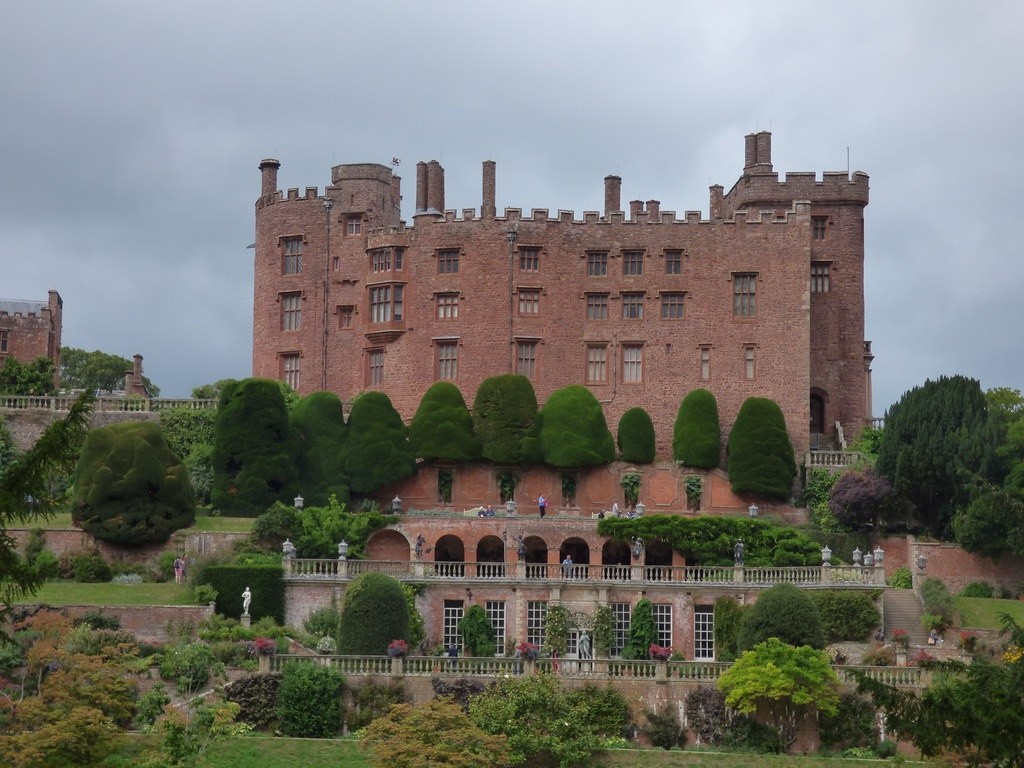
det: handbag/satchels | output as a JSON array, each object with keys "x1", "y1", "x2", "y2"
[{"x1": 544, "y1": 501, "x2": 550, "y2": 507}]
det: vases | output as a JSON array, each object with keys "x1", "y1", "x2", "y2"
[
  {"x1": 322, "y1": 651, "x2": 331, "y2": 655},
  {"x1": 965, "y1": 643, "x2": 975, "y2": 651},
  {"x1": 897, "y1": 639, "x2": 902, "y2": 644}
]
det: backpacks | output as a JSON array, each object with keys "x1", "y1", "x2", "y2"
[
  {"x1": 875, "y1": 633, "x2": 880, "y2": 640},
  {"x1": 175, "y1": 560, "x2": 180, "y2": 568}
]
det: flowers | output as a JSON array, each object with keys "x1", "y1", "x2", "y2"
[
  {"x1": 959, "y1": 631, "x2": 981, "y2": 648},
  {"x1": 317, "y1": 635, "x2": 336, "y2": 654},
  {"x1": 387, "y1": 639, "x2": 408, "y2": 656},
  {"x1": 255, "y1": 634, "x2": 275, "y2": 654},
  {"x1": 515, "y1": 640, "x2": 539, "y2": 659},
  {"x1": 891, "y1": 628, "x2": 911, "y2": 642},
  {"x1": 544, "y1": 604, "x2": 570, "y2": 656},
  {"x1": 591, "y1": 606, "x2": 616, "y2": 656},
  {"x1": 650, "y1": 643, "x2": 673, "y2": 661}
]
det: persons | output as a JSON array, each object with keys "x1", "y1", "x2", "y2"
[
  {"x1": 927, "y1": 629, "x2": 939, "y2": 645},
  {"x1": 598, "y1": 502, "x2": 636, "y2": 520},
  {"x1": 628, "y1": 535, "x2": 642, "y2": 560},
  {"x1": 577, "y1": 631, "x2": 592, "y2": 661},
  {"x1": 734, "y1": 538, "x2": 744, "y2": 566},
  {"x1": 478, "y1": 504, "x2": 487, "y2": 517},
  {"x1": 446, "y1": 643, "x2": 459, "y2": 671},
  {"x1": 874, "y1": 628, "x2": 885, "y2": 643},
  {"x1": 242, "y1": 586, "x2": 252, "y2": 614},
  {"x1": 173, "y1": 554, "x2": 190, "y2": 583},
  {"x1": 512, "y1": 534, "x2": 526, "y2": 560},
  {"x1": 560, "y1": 554, "x2": 574, "y2": 579},
  {"x1": 538, "y1": 492, "x2": 547, "y2": 519},
  {"x1": 487, "y1": 504, "x2": 496, "y2": 518},
  {"x1": 415, "y1": 533, "x2": 425, "y2": 557}
]
